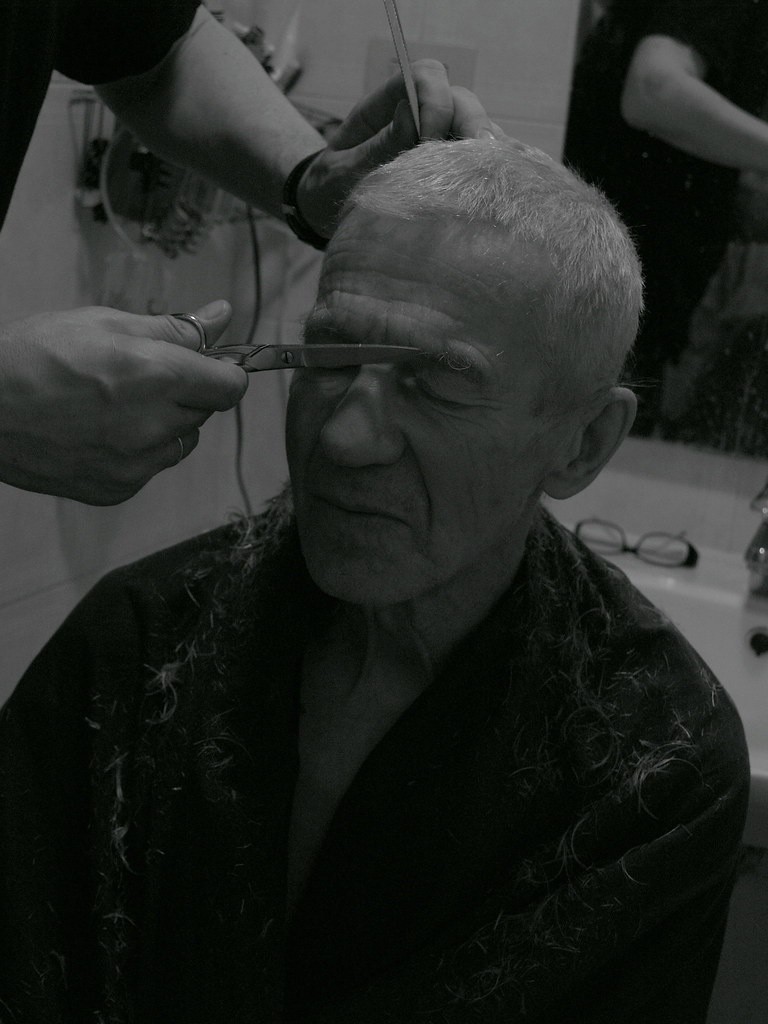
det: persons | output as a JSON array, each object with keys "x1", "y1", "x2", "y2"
[
  {"x1": 0, "y1": 0, "x2": 510, "y2": 507},
  {"x1": 564, "y1": 0, "x2": 768, "y2": 436},
  {"x1": 0, "y1": 137, "x2": 749, "y2": 1024}
]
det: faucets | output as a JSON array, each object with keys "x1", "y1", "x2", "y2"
[{"x1": 744, "y1": 480, "x2": 768, "y2": 606}]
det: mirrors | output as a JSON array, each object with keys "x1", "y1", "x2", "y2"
[{"x1": 562, "y1": 0, "x2": 767, "y2": 460}]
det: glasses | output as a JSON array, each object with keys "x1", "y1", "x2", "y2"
[{"x1": 574, "y1": 519, "x2": 698, "y2": 569}]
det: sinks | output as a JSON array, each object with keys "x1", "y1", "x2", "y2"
[{"x1": 550, "y1": 519, "x2": 768, "y2": 857}]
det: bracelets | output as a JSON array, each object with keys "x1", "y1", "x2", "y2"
[{"x1": 283, "y1": 149, "x2": 330, "y2": 251}]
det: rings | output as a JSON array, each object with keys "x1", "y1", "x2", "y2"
[{"x1": 171, "y1": 437, "x2": 183, "y2": 465}]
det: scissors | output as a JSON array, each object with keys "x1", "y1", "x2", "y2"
[{"x1": 166, "y1": 308, "x2": 433, "y2": 376}]
